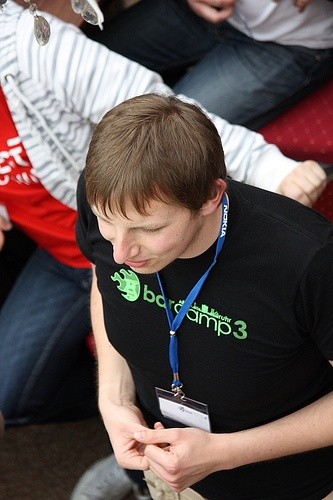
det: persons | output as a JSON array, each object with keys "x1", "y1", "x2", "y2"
[
  {"x1": 0, "y1": 0, "x2": 333, "y2": 424},
  {"x1": 70, "y1": 93, "x2": 333, "y2": 500}
]
